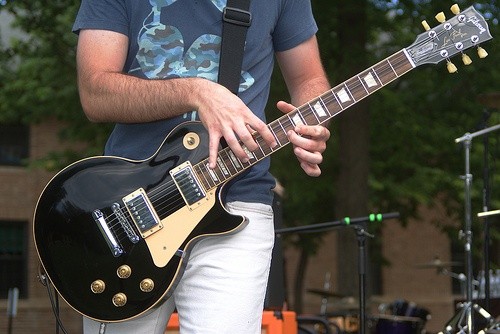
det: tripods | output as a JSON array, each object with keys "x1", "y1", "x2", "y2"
[{"x1": 437, "y1": 124, "x2": 500, "y2": 334}]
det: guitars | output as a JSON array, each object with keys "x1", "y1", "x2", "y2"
[{"x1": 32, "y1": 3, "x2": 493, "y2": 323}]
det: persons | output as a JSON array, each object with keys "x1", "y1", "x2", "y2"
[{"x1": 76, "y1": 0, "x2": 332, "y2": 334}]
